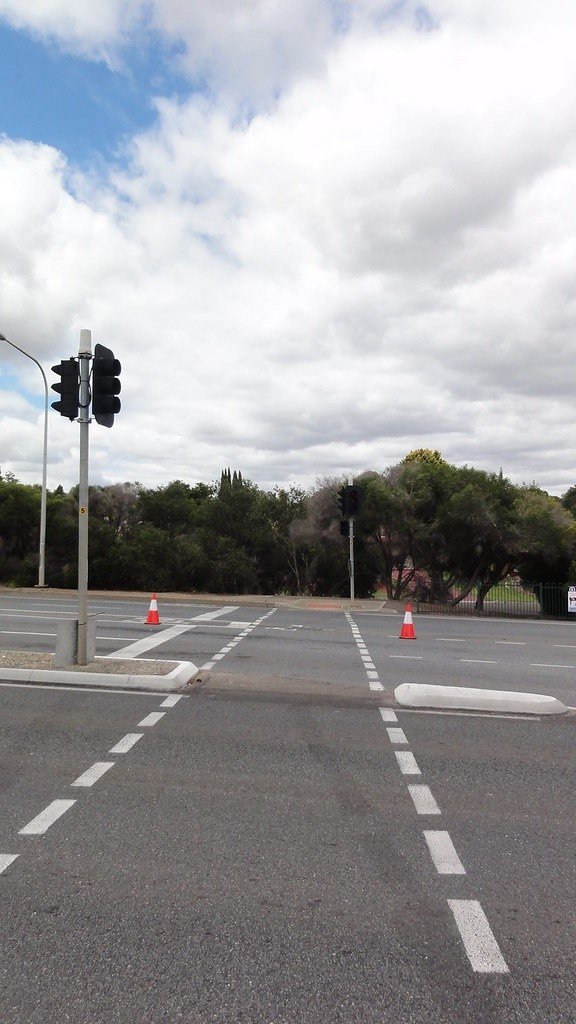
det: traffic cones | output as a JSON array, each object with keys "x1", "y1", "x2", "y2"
[
  {"x1": 399, "y1": 603, "x2": 416, "y2": 639},
  {"x1": 144, "y1": 593, "x2": 161, "y2": 624}
]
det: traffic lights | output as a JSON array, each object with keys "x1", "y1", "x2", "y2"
[
  {"x1": 51, "y1": 343, "x2": 122, "y2": 428},
  {"x1": 337, "y1": 484, "x2": 364, "y2": 517}
]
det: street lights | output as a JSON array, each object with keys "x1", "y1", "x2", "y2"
[{"x1": 0, "y1": 330, "x2": 49, "y2": 588}]
